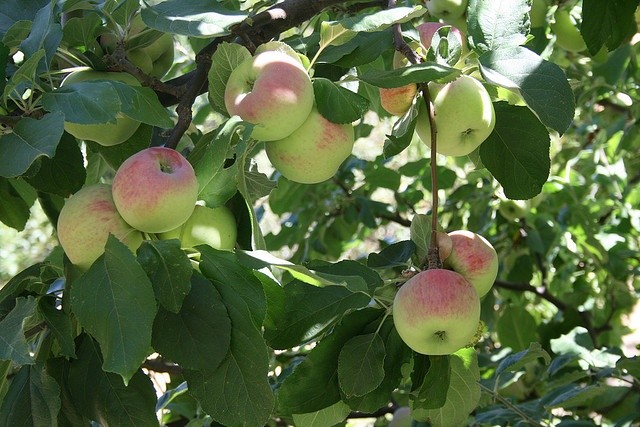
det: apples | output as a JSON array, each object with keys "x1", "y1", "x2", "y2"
[
  {"x1": 393, "y1": 21, "x2": 469, "y2": 70},
  {"x1": 414, "y1": 75, "x2": 496, "y2": 157},
  {"x1": 57, "y1": 68, "x2": 143, "y2": 146},
  {"x1": 57, "y1": 182, "x2": 135, "y2": 270},
  {"x1": 266, "y1": 107, "x2": 354, "y2": 184},
  {"x1": 99, "y1": 9, "x2": 175, "y2": 81},
  {"x1": 377, "y1": 86, "x2": 416, "y2": 115},
  {"x1": 392, "y1": 270, "x2": 481, "y2": 357},
  {"x1": 112, "y1": 147, "x2": 198, "y2": 235},
  {"x1": 226, "y1": 52, "x2": 316, "y2": 141},
  {"x1": 447, "y1": 230, "x2": 499, "y2": 299}
]
